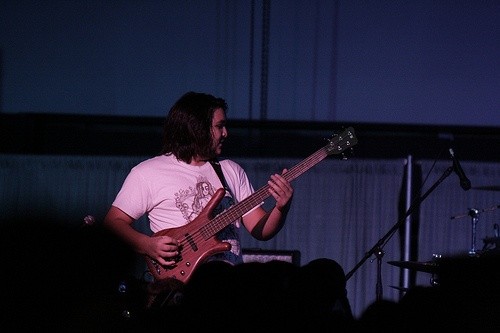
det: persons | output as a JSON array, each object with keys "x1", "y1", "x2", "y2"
[{"x1": 105, "y1": 92, "x2": 295, "y2": 271}]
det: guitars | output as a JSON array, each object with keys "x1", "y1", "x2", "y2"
[{"x1": 145, "y1": 124, "x2": 358, "y2": 283}]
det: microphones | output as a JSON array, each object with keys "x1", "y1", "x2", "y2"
[
  {"x1": 79, "y1": 215, "x2": 96, "y2": 227},
  {"x1": 449, "y1": 148, "x2": 471, "y2": 191}
]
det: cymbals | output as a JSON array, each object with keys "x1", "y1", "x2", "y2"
[{"x1": 387, "y1": 258, "x2": 440, "y2": 276}]
